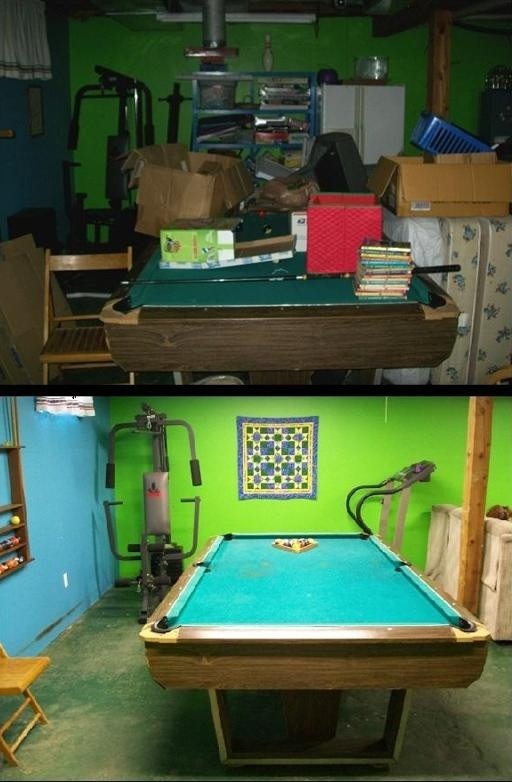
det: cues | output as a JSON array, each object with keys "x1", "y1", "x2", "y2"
[{"x1": 120, "y1": 263, "x2": 461, "y2": 286}]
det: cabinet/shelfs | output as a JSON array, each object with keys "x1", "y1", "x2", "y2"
[{"x1": 176, "y1": 74, "x2": 320, "y2": 175}]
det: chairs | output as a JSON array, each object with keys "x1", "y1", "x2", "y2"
[
  {"x1": 34, "y1": 245, "x2": 136, "y2": 386},
  {"x1": 0, "y1": 634, "x2": 52, "y2": 770}
]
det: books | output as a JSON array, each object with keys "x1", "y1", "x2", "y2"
[{"x1": 355, "y1": 240, "x2": 413, "y2": 302}]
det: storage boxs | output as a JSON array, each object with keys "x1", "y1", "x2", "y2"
[
  {"x1": 115, "y1": 145, "x2": 254, "y2": 237},
  {"x1": 366, "y1": 149, "x2": 512, "y2": 219}
]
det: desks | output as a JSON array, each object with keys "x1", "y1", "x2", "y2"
[
  {"x1": 102, "y1": 225, "x2": 466, "y2": 385},
  {"x1": 137, "y1": 531, "x2": 493, "y2": 772}
]
